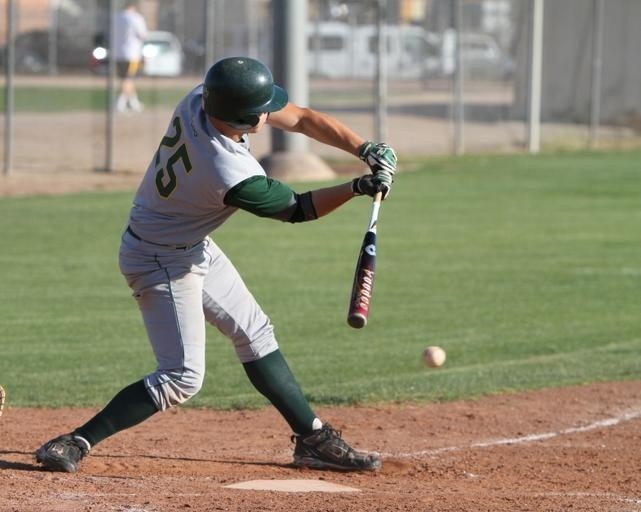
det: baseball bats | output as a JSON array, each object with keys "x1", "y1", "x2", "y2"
[{"x1": 347, "y1": 192, "x2": 382, "y2": 328}]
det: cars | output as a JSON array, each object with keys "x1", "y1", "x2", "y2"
[
  {"x1": 306, "y1": 19, "x2": 499, "y2": 78},
  {"x1": 0, "y1": 29, "x2": 102, "y2": 69},
  {"x1": 139, "y1": 32, "x2": 184, "y2": 78}
]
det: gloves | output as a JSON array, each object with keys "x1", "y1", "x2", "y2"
[
  {"x1": 349, "y1": 167, "x2": 392, "y2": 200},
  {"x1": 359, "y1": 139, "x2": 397, "y2": 174}
]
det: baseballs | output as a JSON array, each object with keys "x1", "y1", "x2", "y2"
[{"x1": 423, "y1": 346, "x2": 445, "y2": 367}]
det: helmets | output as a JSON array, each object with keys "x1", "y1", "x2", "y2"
[{"x1": 199, "y1": 54, "x2": 291, "y2": 131}]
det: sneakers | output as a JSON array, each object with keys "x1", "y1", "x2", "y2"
[
  {"x1": 289, "y1": 423, "x2": 381, "y2": 474},
  {"x1": 33, "y1": 429, "x2": 91, "y2": 474}
]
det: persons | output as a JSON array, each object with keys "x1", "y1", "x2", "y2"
[
  {"x1": 115, "y1": 1, "x2": 151, "y2": 117},
  {"x1": 34, "y1": 55, "x2": 397, "y2": 474}
]
